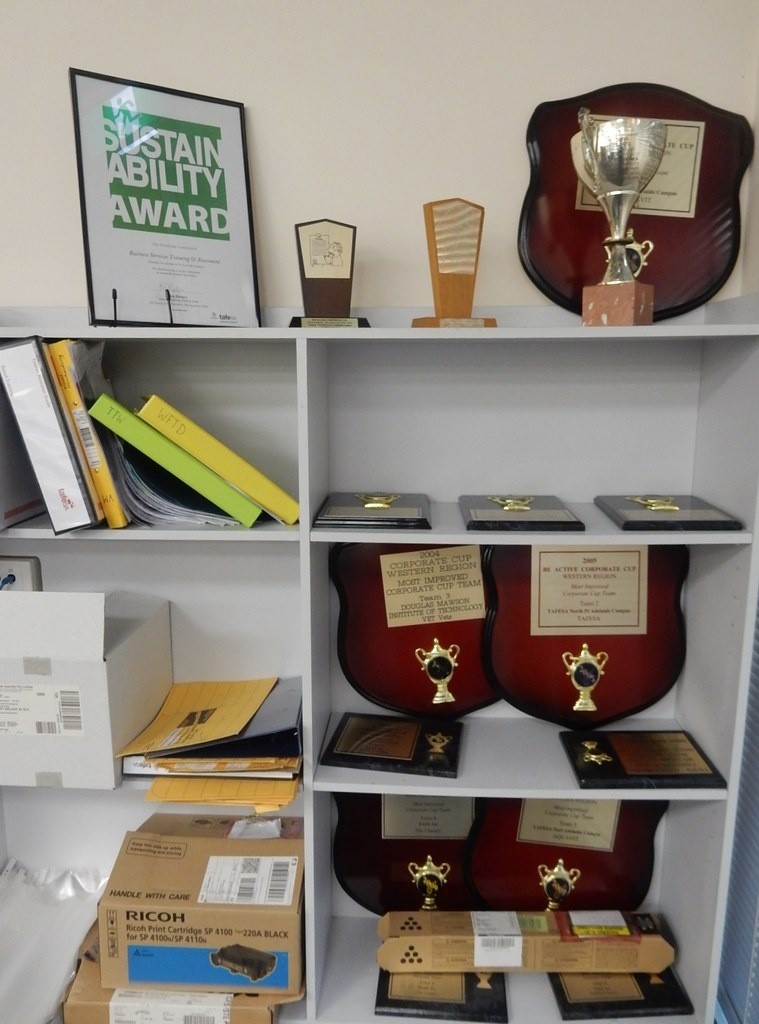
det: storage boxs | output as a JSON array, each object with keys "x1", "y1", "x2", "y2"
[
  {"x1": 98, "y1": 815, "x2": 305, "y2": 999},
  {"x1": 1, "y1": 590, "x2": 173, "y2": 790},
  {"x1": 1, "y1": 336, "x2": 105, "y2": 535},
  {"x1": 64, "y1": 922, "x2": 279, "y2": 1023},
  {"x1": 376, "y1": 910, "x2": 678, "y2": 972}
]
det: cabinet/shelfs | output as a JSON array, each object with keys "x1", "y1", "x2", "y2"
[{"x1": 0, "y1": 303, "x2": 758, "y2": 1024}]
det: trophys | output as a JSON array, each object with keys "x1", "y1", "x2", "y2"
[{"x1": 577, "y1": 107, "x2": 669, "y2": 328}]
